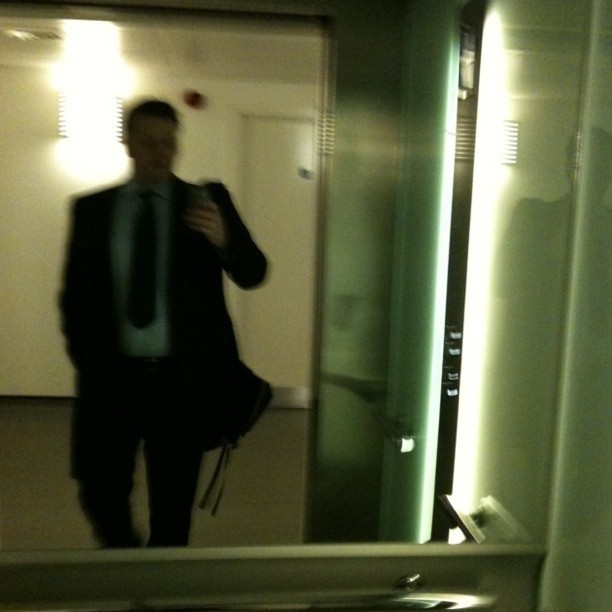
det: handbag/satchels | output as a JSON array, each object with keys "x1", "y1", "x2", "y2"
[{"x1": 168, "y1": 186, "x2": 272, "y2": 517}]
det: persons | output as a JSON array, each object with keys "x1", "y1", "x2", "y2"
[{"x1": 56, "y1": 99, "x2": 274, "y2": 545}]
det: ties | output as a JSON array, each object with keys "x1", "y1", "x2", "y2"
[{"x1": 124, "y1": 190, "x2": 156, "y2": 332}]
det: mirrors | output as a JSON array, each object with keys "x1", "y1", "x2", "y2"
[{"x1": 0, "y1": 0, "x2": 597, "y2": 551}]
